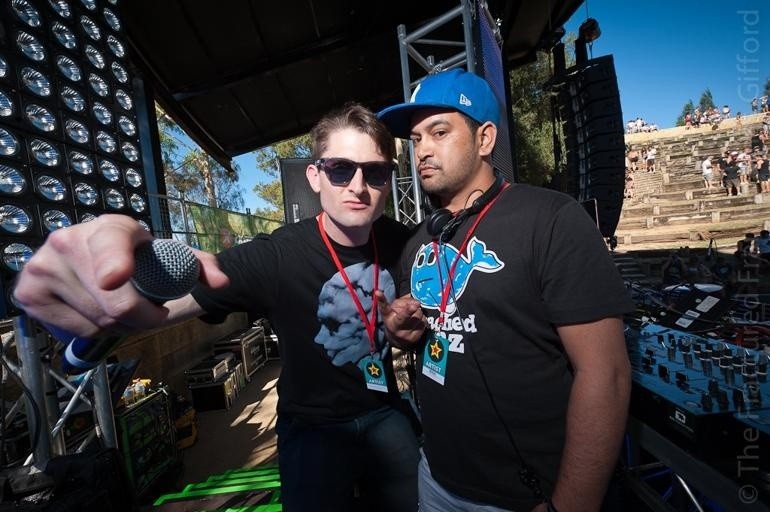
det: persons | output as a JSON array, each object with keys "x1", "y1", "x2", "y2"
[
  {"x1": 10, "y1": 99, "x2": 428, "y2": 512},
  {"x1": 618, "y1": 93, "x2": 770, "y2": 195},
  {"x1": 658, "y1": 230, "x2": 770, "y2": 313},
  {"x1": 374, "y1": 67, "x2": 645, "y2": 511}
]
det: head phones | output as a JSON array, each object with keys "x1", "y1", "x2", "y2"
[{"x1": 421, "y1": 166, "x2": 504, "y2": 239}]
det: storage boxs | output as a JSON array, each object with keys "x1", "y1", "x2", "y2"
[
  {"x1": 189, "y1": 361, "x2": 245, "y2": 413},
  {"x1": 184, "y1": 353, "x2": 237, "y2": 383},
  {"x1": 215, "y1": 327, "x2": 268, "y2": 382},
  {"x1": 263, "y1": 327, "x2": 281, "y2": 361}
]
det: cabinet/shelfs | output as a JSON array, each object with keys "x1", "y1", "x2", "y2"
[{"x1": 113, "y1": 384, "x2": 182, "y2": 506}]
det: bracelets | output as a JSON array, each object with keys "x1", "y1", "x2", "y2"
[{"x1": 546, "y1": 500, "x2": 560, "y2": 512}]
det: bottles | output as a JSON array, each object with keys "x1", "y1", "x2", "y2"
[
  {"x1": 124, "y1": 383, "x2": 137, "y2": 408},
  {"x1": 135, "y1": 378, "x2": 145, "y2": 401}
]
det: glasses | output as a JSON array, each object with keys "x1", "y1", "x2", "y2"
[{"x1": 311, "y1": 155, "x2": 403, "y2": 187}]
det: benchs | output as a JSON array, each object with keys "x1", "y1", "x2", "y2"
[{"x1": 622, "y1": 120, "x2": 769, "y2": 244}]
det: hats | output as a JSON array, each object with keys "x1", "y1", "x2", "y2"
[{"x1": 372, "y1": 66, "x2": 506, "y2": 134}]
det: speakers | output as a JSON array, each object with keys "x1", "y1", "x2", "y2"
[
  {"x1": 279, "y1": 158, "x2": 323, "y2": 224},
  {"x1": 553, "y1": 52, "x2": 627, "y2": 237}
]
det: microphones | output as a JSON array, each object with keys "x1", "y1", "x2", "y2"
[{"x1": 61, "y1": 240, "x2": 201, "y2": 376}]
